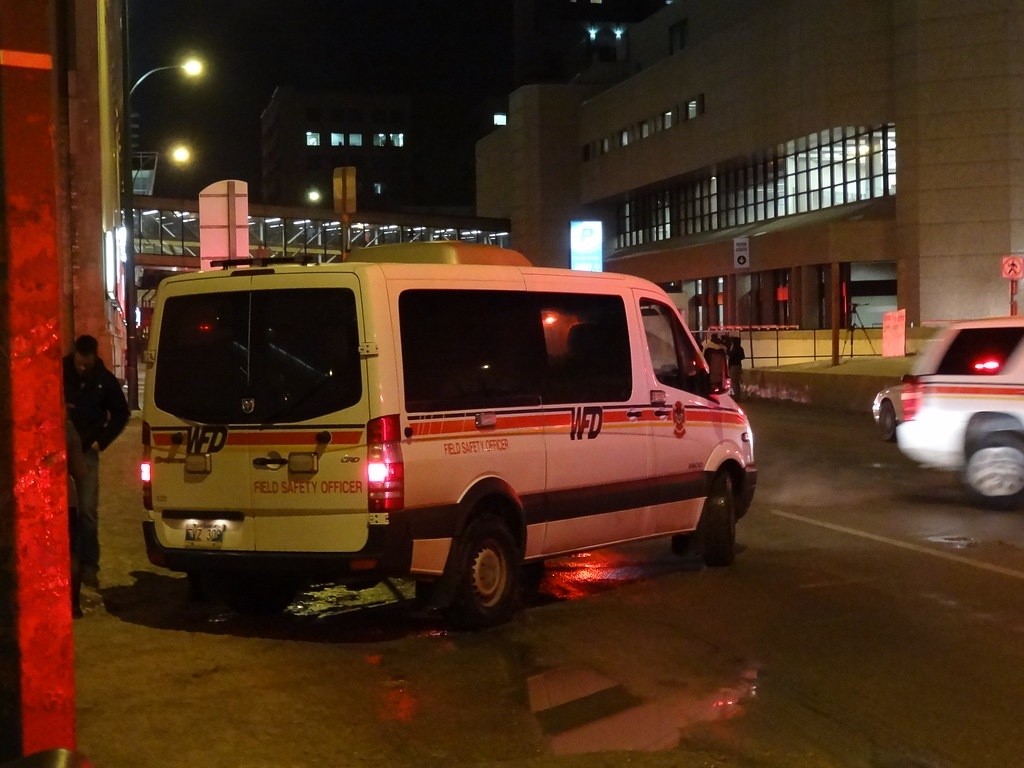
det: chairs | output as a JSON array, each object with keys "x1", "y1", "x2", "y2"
[{"x1": 549, "y1": 321, "x2": 631, "y2": 399}]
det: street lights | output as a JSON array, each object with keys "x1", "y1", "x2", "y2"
[{"x1": 124, "y1": 59, "x2": 200, "y2": 410}]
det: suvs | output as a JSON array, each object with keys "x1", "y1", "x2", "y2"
[{"x1": 874, "y1": 316, "x2": 1024, "y2": 443}]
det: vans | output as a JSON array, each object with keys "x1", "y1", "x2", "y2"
[{"x1": 142, "y1": 242, "x2": 757, "y2": 631}]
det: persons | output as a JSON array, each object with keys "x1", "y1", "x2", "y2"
[
  {"x1": 63, "y1": 334, "x2": 131, "y2": 616},
  {"x1": 702, "y1": 329, "x2": 745, "y2": 399}
]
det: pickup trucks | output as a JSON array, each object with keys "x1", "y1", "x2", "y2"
[{"x1": 897, "y1": 340, "x2": 1024, "y2": 510}]
